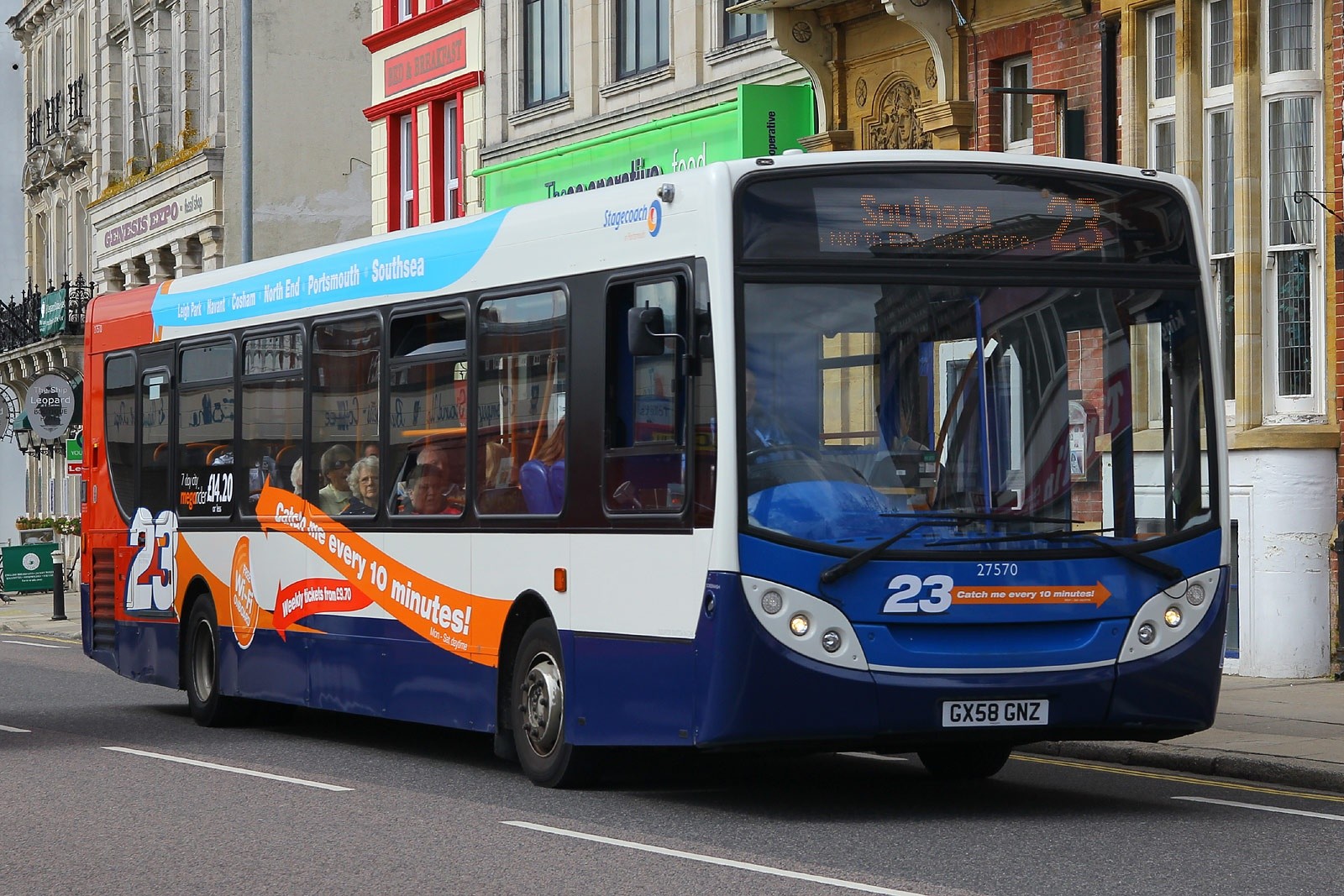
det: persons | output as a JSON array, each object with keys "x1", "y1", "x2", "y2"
[
  {"x1": 822, "y1": 399, "x2": 940, "y2": 511},
  {"x1": 694, "y1": 366, "x2": 815, "y2": 492},
  {"x1": 141, "y1": 415, "x2": 566, "y2": 515}
]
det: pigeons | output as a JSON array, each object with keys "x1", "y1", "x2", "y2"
[{"x1": 0, "y1": 593, "x2": 16, "y2": 605}]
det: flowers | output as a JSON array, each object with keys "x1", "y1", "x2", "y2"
[{"x1": 60, "y1": 520, "x2": 74, "y2": 534}]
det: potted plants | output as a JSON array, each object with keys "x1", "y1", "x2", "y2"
[{"x1": 15, "y1": 517, "x2": 81, "y2": 535}]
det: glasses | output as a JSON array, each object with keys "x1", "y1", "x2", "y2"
[
  {"x1": 358, "y1": 477, "x2": 379, "y2": 483},
  {"x1": 330, "y1": 460, "x2": 356, "y2": 470}
]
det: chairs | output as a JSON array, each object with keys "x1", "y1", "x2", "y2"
[{"x1": 151, "y1": 428, "x2": 525, "y2": 516}]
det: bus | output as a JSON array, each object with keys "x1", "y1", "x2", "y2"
[{"x1": 74, "y1": 145, "x2": 1232, "y2": 792}]
